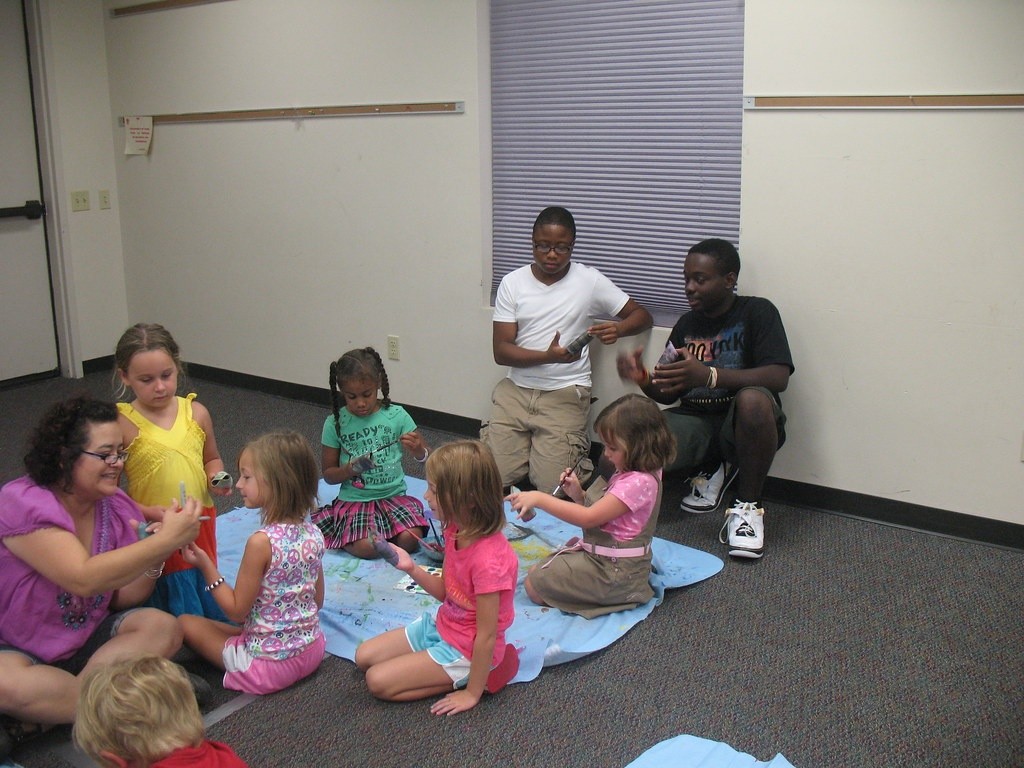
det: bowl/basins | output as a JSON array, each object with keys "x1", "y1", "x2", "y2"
[{"x1": 419, "y1": 536, "x2": 444, "y2": 563}]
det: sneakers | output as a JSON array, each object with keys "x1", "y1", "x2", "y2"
[
  {"x1": 679, "y1": 459, "x2": 740, "y2": 514},
  {"x1": 719, "y1": 497, "x2": 765, "y2": 558}
]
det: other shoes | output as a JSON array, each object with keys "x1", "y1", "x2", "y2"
[
  {"x1": 0, "y1": 714, "x2": 44, "y2": 762},
  {"x1": 482, "y1": 642, "x2": 519, "y2": 694},
  {"x1": 187, "y1": 673, "x2": 214, "y2": 706}
]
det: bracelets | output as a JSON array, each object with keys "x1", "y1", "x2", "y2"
[
  {"x1": 705, "y1": 366, "x2": 718, "y2": 389},
  {"x1": 204, "y1": 576, "x2": 225, "y2": 592},
  {"x1": 145, "y1": 562, "x2": 165, "y2": 578},
  {"x1": 414, "y1": 447, "x2": 429, "y2": 463}
]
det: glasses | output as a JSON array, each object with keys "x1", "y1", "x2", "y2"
[
  {"x1": 532, "y1": 238, "x2": 575, "y2": 254},
  {"x1": 80, "y1": 449, "x2": 130, "y2": 464}
]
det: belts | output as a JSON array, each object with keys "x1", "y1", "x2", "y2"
[{"x1": 541, "y1": 536, "x2": 655, "y2": 569}]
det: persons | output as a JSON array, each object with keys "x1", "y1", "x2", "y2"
[
  {"x1": 481, "y1": 205, "x2": 654, "y2": 499},
  {"x1": 177, "y1": 429, "x2": 326, "y2": 693},
  {"x1": 71, "y1": 650, "x2": 248, "y2": 768},
  {"x1": 0, "y1": 397, "x2": 202, "y2": 743},
  {"x1": 310, "y1": 347, "x2": 432, "y2": 560},
  {"x1": 616, "y1": 238, "x2": 796, "y2": 559},
  {"x1": 111, "y1": 322, "x2": 233, "y2": 623},
  {"x1": 355, "y1": 438, "x2": 518, "y2": 717},
  {"x1": 503, "y1": 393, "x2": 677, "y2": 619}
]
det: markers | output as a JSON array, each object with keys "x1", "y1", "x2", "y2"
[{"x1": 179, "y1": 480, "x2": 186, "y2": 508}]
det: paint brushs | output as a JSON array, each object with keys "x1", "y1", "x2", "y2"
[
  {"x1": 403, "y1": 526, "x2": 436, "y2": 550},
  {"x1": 427, "y1": 517, "x2": 441, "y2": 546},
  {"x1": 553, "y1": 449, "x2": 591, "y2": 497},
  {"x1": 374, "y1": 439, "x2": 400, "y2": 454}
]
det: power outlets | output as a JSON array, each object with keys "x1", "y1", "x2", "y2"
[
  {"x1": 387, "y1": 335, "x2": 400, "y2": 361},
  {"x1": 71, "y1": 190, "x2": 88, "y2": 212}
]
